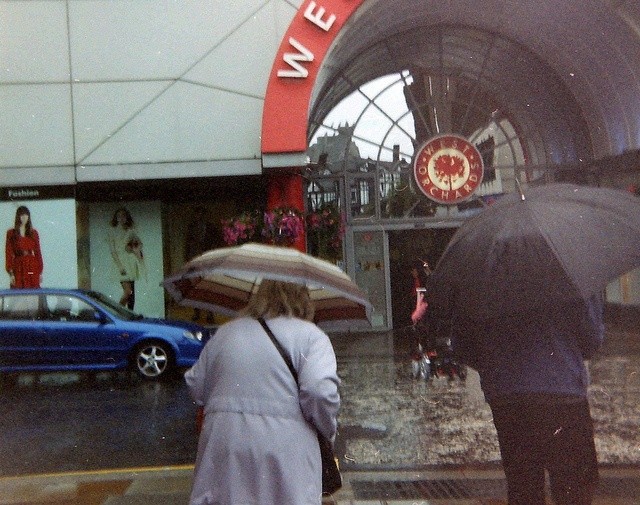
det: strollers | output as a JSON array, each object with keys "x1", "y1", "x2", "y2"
[{"x1": 412, "y1": 287, "x2": 467, "y2": 382}]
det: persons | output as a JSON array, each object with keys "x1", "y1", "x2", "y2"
[
  {"x1": 5, "y1": 204, "x2": 43, "y2": 289},
  {"x1": 451, "y1": 286, "x2": 607, "y2": 505},
  {"x1": 183, "y1": 277, "x2": 341, "y2": 505},
  {"x1": 106, "y1": 206, "x2": 145, "y2": 310}
]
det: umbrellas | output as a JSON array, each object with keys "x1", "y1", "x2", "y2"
[
  {"x1": 418, "y1": 176, "x2": 639, "y2": 324},
  {"x1": 159, "y1": 242, "x2": 376, "y2": 327}
]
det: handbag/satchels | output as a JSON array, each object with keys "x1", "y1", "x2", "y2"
[{"x1": 316, "y1": 431, "x2": 343, "y2": 498}]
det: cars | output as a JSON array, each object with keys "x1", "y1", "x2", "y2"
[{"x1": 0, "y1": 288, "x2": 210, "y2": 380}]
